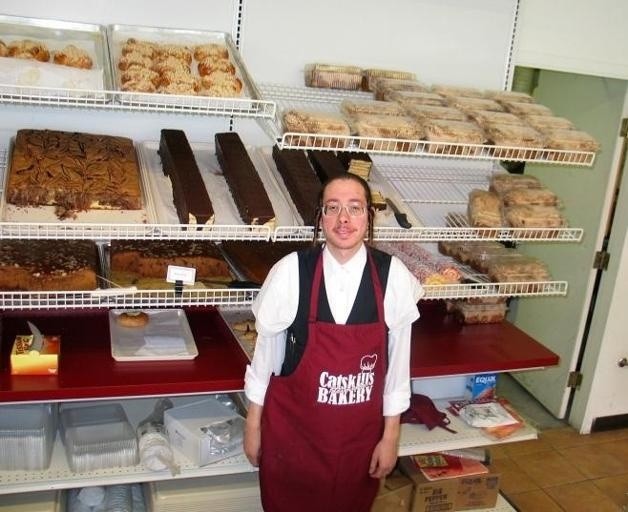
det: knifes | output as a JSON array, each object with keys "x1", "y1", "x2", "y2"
[{"x1": 386, "y1": 198, "x2": 411, "y2": 228}]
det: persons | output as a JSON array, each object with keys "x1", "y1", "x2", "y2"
[{"x1": 244, "y1": 172, "x2": 421, "y2": 512}]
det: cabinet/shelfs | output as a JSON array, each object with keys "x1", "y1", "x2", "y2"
[
  {"x1": 504, "y1": 66, "x2": 627, "y2": 435},
  {"x1": 1, "y1": 82, "x2": 596, "y2": 494}
]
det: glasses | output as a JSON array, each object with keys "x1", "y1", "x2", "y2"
[{"x1": 320, "y1": 202, "x2": 369, "y2": 217}]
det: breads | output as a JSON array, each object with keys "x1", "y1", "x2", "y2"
[{"x1": 0, "y1": 37, "x2": 601, "y2": 357}]
